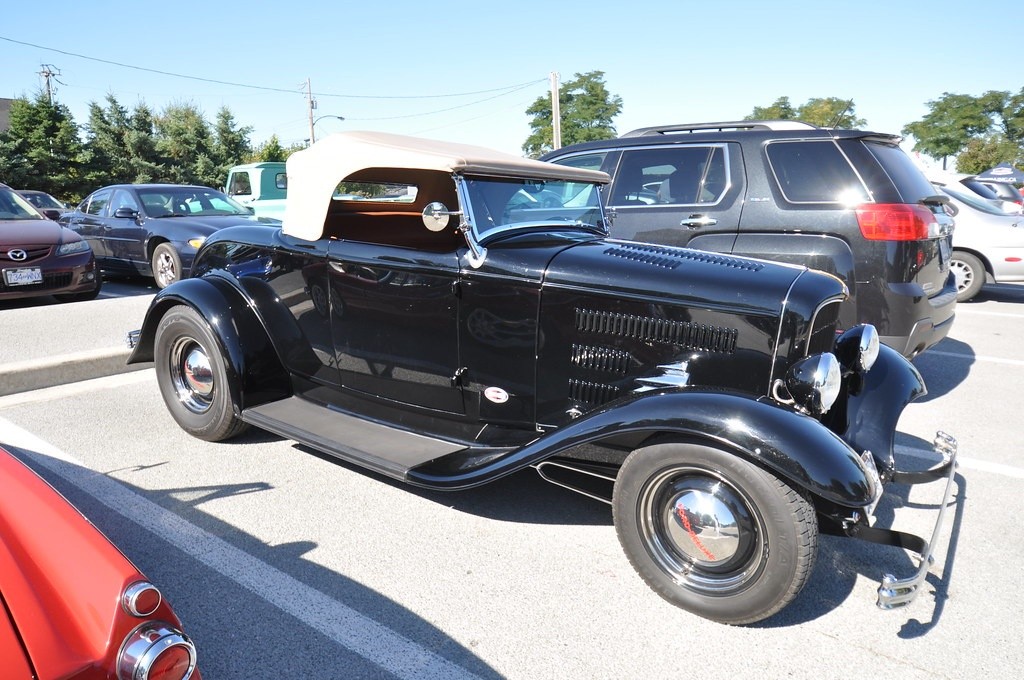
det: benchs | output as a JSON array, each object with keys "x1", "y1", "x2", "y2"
[{"x1": 326, "y1": 210, "x2": 465, "y2": 253}]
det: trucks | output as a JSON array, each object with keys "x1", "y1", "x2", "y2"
[{"x1": 225, "y1": 162, "x2": 416, "y2": 227}]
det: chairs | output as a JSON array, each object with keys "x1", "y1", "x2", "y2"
[
  {"x1": 670, "y1": 169, "x2": 696, "y2": 203},
  {"x1": 145, "y1": 204, "x2": 168, "y2": 217},
  {"x1": 621, "y1": 168, "x2": 648, "y2": 205}
]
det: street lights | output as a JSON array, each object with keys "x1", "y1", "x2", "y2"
[{"x1": 309, "y1": 115, "x2": 345, "y2": 144}]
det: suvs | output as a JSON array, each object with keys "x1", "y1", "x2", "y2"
[{"x1": 501, "y1": 120, "x2": 959, "y2": 360}]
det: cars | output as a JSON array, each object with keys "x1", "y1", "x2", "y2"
[
  {"x1": 0, "y1": 446, "x2": 202, "y2": 680},
  {"x1": 14, "y1": 190, "x2": 71, "y2": 217},
  {"x1": 55, "y1": 184, "x2": 271, "y2": 289},
  {"x1": 929, "y1": 174, "x2": 1024, "y2": 303},
  {"x1": 0, "y1": 183, "x2": 102, "y2": 301},
  {"x1": 126, "y1": 133, "x2": 960, "y2": 626}
]
